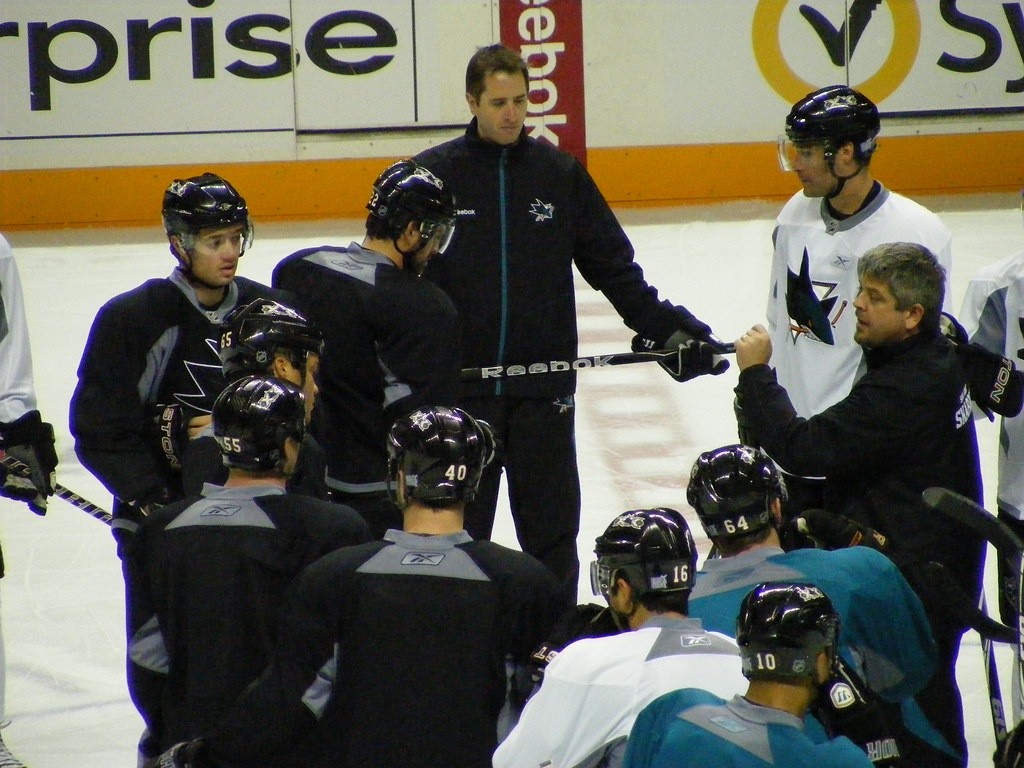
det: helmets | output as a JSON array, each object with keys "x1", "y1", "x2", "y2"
[
  {"x1": 160, "y1": 172, "x2": 252, "y2": 233},
  {"x1": 594, "y1": 507, "x2": 698, "y2": 598},
  {"x1": 367, "y1": 157, "x2": 457, "y2": 228},
  {"x1": 784, "y1": 84, "x2": 880, "y2": 164},
  {"x1": 220, "y1": 298, "x2": 332, "y2": 385},
  {"x1": 686, "y1": 444, "x2": 789, "y2": 546},
  {"x1": 211, "y1": 374, "x2": 309, "y2": 470},
  {"x1": 735, "y1": 579, "x2": 840, "y2": 688},
  {"x1": 385, "y1": 405, "x2": 499, "y2": 501}
]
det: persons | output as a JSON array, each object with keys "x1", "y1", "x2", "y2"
[
  {"x1": 764, "y1": 84, "x2": 957, "y2": 551},
  {"x1": 0, "y1": 232, "x2": 60, "y2": 768},
  {"x1": 409, "y1": 44, "x2": 728, "y2": 606},
  {"x1": 69, "y1": 156, "x2": 1024, "y2": 767}
]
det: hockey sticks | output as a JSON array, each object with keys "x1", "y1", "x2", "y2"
[
  {"x1": 0, "y1": 448, "x2": 116, "y2": 524},
  {"x1": 919, "y1": 485, "x2": 1024, "y2": 767},
  {"x1": 456, "y1": 340, "x2": 741, "y2": 380},
  {"x1": 974, "y1": 583, "x2": 1006, "y2": 755}
]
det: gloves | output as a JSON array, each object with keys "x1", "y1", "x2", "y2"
[
  {"x1": 126, "y1": 484, "x2": 191, "y2": 518},
  {"x1": 631, "y1": 303, "x2": 730, "y2": 382},
  {"x1": 529, "y1": 604, "x2": 630, "y2": 687},
  {"x1": 809, "y1": 647, "x2": 901, "y2": 767},
  {"x1": 791, "y1": 509, "x2": 890, "y2": 558},
  {"x1": 0, "y1": 409, "x2": 60, "y2": 517},
  {"x1": 950, "y1": 333, "x2": 1024, "y2": 418}
]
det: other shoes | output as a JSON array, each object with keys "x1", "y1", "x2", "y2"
[{"x1": 0, "y1": 722, "x2": 26, "y2": 768}]
